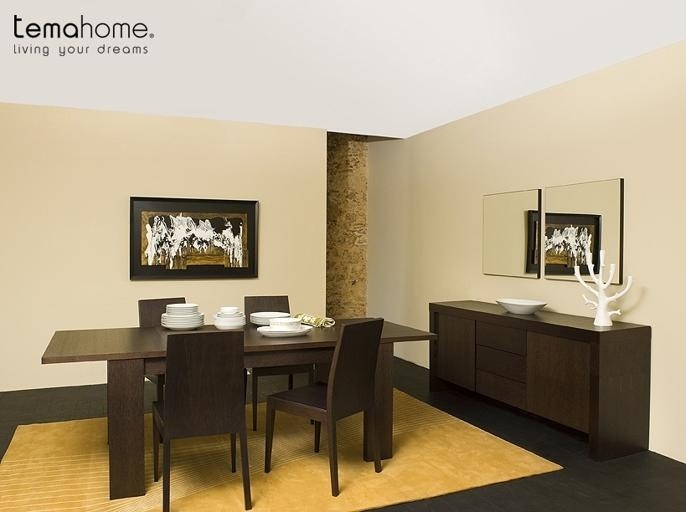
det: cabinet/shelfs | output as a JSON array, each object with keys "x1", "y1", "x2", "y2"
[{"x1": 429, "y1": 301, "x2": 651, "y2": 459}]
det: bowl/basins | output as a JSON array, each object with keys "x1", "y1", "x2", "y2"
[
  {"x1": 220, "y1": 306, "x2": 239, "y2": 314},
  {"x1": 496, "y1": 298, "x2": 548, "y2": 315}
]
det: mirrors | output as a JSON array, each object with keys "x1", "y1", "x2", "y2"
[
  {"x1": 545, "y1": 177, "x2": 622, "y2": 286},
  {"x1": 482, "y1": 190, "x2": 541, "y2": 279}
]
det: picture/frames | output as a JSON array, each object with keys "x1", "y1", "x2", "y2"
[
  {"x1": 129, "y1": 197, "x2": 259, "y2": 281},
  {"x1": 527, "y1": 210, "x2": 601, "y2": 275}
]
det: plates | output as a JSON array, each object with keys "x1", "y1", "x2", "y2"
[
  {"x1": 250, "y1": 311, "x2": 291, "y2": 326},
  {"x1": 213, "y1": 312, "x2": 247, "y2": 331},
  {"x1": 257, "y1": 325, "x2": 313, "y2": 337},
  {"x1": 161, "y1": 303, "x2": 204, "y2": 331},
  {"x1": 270, "y1": 317, "x2": 302, "y2": 326}
]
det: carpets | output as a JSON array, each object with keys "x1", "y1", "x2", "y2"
[{"x1": 0, "y1": 387, "x2": 564, "y2": 512}]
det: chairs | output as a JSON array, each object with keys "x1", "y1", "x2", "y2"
[
  {"x1": 245, "y1": 295, "x2": 314, "y2": 431},
  {"x1": 138, "y1": 297, "x2": 186, "y2": 397},
  {"x1": 152, "y1": 330, "x2": 252, "y2": 512},
  {"x1": 264, "y1": 316, "x2": 385, "y2": 497}
]
